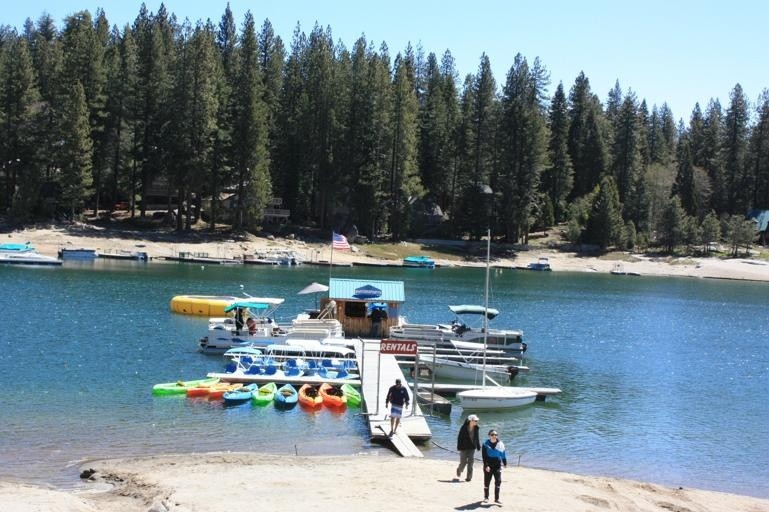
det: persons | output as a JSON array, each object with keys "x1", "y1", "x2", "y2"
[
  {"x1": 386, "y1": 379, "x2": 410, "y2": 434},
  {"x1": 457, "y1": 414, "x2": 480, "y2": 481},
  {"x1": 379, "y1": 307, "x2": 388, "y2": 339},
  {"x1": 367, "y1": 309, "x2": 380, "y2": 338},
  {"x1": 246, "y1": 318, "x2": 256, "y2": 336},
  {"x1": 235, "y1": 308, "x2": 244, "y2": 336},
  {"x1": 482, "y1": 430, "x2": 507, "y2": 507}
]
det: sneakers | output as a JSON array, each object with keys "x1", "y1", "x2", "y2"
[{"x1": 457, "y1": 469, "x2": 461, "y2": 477}]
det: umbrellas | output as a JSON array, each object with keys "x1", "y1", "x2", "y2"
[{"x1": 297, "y1": 282, "x2": 329, "y2": 312}]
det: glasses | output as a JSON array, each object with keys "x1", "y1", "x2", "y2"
[{"x1": 490, "y1": 433, "x2": 497, "y2": 436}]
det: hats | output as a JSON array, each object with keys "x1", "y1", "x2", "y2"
[{"x1": 468, "y1": 415, "x2": 479, "y2": 421}]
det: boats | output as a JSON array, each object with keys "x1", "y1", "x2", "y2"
[
  {"x1": 0, "y1": 242, "x2": 62, "y2": 265},
  {"x1": 389, "y1": 305, "x2": 526, "y2": 356},
  {"x1": 413, "y1": 385, "x2": 452, "y2": 417},
  {"x1": 195, "y1": 297, "x2": 343, "y2": 353},
  {"x1": 611, "y1": 269, "x2": 640, "y2": 277},
  {"x1": 527, "y1": 257, "x2": 550, "y2": 272},
  {"x1": 242, "y1": 251, "x2": 300, "y2": 265},
  {"x1": 170, "y1": 294, "x2": 247, "y2": 314},
  {"x1": 454, "y1": 388, "x2": 537, "y2": 410},
  {"x1": 402, "y1": 256, "x2": 435, "y2": 269},
  {"x1": 151, "y1": 344, "x2": 365, "y2": 410},
  {"x1": 408, "y1": 338, "x2": 530, "y2": 384}
]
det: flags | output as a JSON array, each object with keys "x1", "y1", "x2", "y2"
[{"x1": 332, "y1": 231, "x2": 351, "y2": 250}]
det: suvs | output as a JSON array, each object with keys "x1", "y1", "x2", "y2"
[{"x1": 114, "y1": 202, "x2": 128, "y2": 210}]
